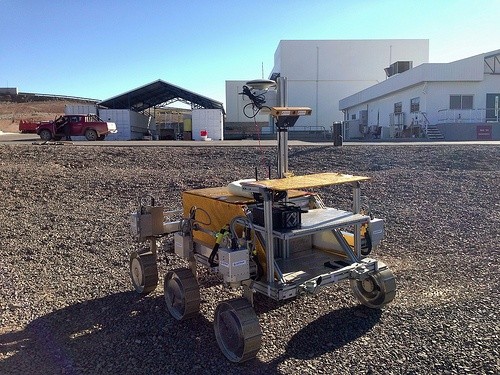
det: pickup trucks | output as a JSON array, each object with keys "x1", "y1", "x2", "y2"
[{"x1": 36, "y1": 113, "x2": 117, "y2": 141}]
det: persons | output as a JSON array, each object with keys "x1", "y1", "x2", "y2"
[{"x1": 61, "y1": 117, "x2": 72, "y2": 141}]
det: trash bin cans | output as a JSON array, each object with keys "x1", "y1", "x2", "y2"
[{"x1": 332, "y1": 120, "x2": 343, "y2": 146}]
